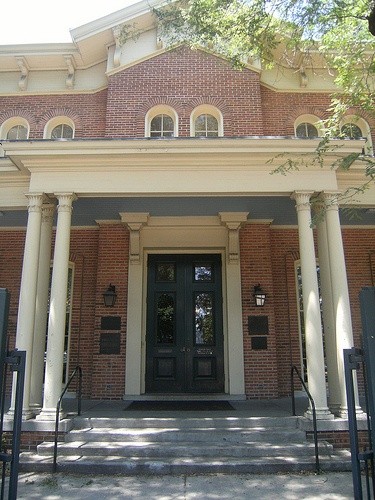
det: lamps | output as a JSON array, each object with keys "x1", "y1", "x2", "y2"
[
  {"x1": 253, "y1": 283, "x2": 266, "y2": 307},
  {"x1": 103, "y1": 284, "x2": 116, "y2": 307}
]
